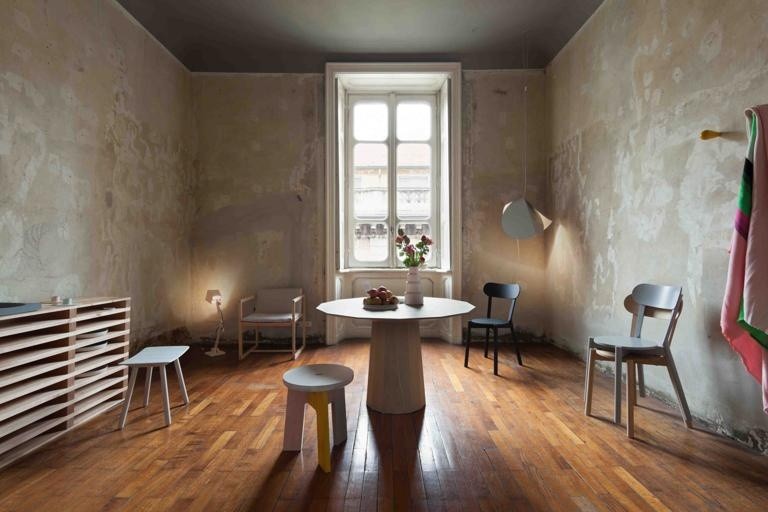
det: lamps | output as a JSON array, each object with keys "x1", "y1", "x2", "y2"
[
  {"x1": 205, "y1": 289, "x2": 227, "y2": 358},
  {"x1": 501, "y1": 84, "x2": 553, "y2": 240}
]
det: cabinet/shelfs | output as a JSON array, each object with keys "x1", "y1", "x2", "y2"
[{"x1": 0, "y1": 295, "x2": 133, "y2": 468}]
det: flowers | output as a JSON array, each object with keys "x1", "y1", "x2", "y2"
[{"x1": 396, "y1": 228, "x2": 434, "y2": 267}]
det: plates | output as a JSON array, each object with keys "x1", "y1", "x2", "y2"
[{"x1": 364, "y1": 305, "x2": 397, "y2": 310}]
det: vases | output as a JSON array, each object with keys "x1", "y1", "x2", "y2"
[{"x1": 404, "y1": 268, "x2": 423, "y2": 306}]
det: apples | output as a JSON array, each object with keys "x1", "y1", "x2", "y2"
[{"x1": 363, "y1": 286, "x2": 398, "y2": 305}]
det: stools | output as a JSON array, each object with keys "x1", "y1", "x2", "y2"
[
  {"x1": 118, "y1": 345, "x2": 190, "y2": 430},
  {"x1": 282, "y1": 363, "x2": 354, "y2": 474}
]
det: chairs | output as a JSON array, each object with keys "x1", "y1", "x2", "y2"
[
  {"x1": 464, "y1": 282, "x2": 522, "y2": 375},
  {"x1": 238, "y1": 289, "x2": 307, "y2": 361},
  {"x1": 584, "y1": 283, "x2": 693, "y2": 439}
]
